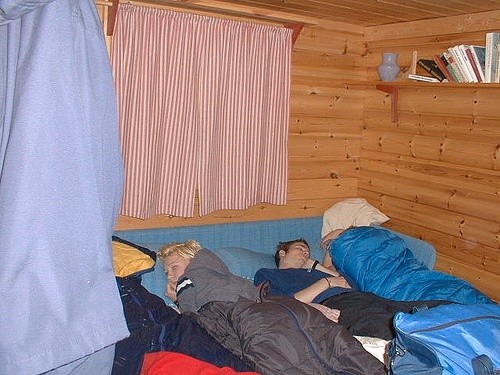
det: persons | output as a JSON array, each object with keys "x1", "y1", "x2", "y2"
[
  {"x1": 0, "y1": 1, "x2": 131, "y2": 375},
  {"x1": 156, "y1": 241, "x2": 261, "y2": 314},
  {"x1": 253, "y1": 226, "x2": 355, "y2": 304}
]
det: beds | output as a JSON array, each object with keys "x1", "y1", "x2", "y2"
[{"x1": 113, "y1": 214, "x2": 437, "y2": 312}]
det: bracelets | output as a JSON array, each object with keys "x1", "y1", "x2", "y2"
[
  {"x1": 325, "y1": 277, "x2": 331, "y2": 287},
  {"x1": 173, "y1": 300, "x2": 178, "y2": 304},
  {"x1": 312, "y1": 261, "x2": 319, "y2": 271}
]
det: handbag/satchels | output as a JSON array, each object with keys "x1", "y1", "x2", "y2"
[{"x1": 387, "y1": 303, "x2": 500, "y2": 375}]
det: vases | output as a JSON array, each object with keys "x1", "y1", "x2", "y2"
[{"x1": 376, "y1": 52, "x2": 401, "y2": 83}]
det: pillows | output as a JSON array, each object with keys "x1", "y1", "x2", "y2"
[{"x1": 211, "y1": 246, "x2": 278, "y2": 281}]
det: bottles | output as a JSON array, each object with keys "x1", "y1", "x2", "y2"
[{"x1": 377, "y1": 52, "x2": 401, "y2": 82}]
